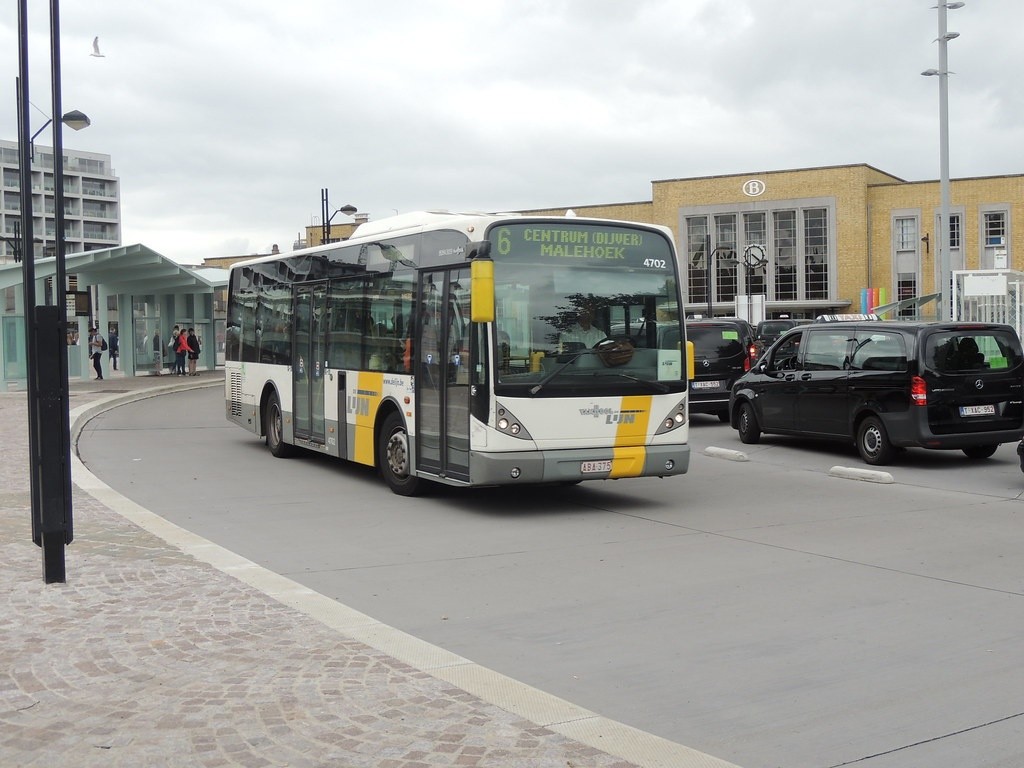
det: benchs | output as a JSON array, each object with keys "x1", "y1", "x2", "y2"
[{"x1": 138, "y1": 361, "x2": 178, "y2": 375}]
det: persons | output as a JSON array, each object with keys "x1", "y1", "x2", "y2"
[
  {"x1": 234, "y1": 304, "x2": 471, "y2": 383},
  {"x1": 65, "y1": 326, "x2": 204, "y2": 377},
  {"x1": 556, "y1": 298, "x2": 608, "y2": 354}
]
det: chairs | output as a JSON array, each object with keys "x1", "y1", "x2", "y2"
[
  {"x1": 232, "y1": 297, "x2": 379, "y2": 330},
  {"x1": 958, "y1": 337, "x2": 985, "y2": 367}
]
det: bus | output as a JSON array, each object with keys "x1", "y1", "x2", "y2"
[{"x1": 226, "y1": 212, "x2": 694, "y2": 497}]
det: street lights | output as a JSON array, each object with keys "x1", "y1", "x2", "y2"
[
  {"x1": 921, "y1": 0, "x2": 965, "y2": 323},
  {"x1": 321, "y1": 188, "x2": 357, "y2": 246},
  {"x1": 15, "y1": 0, "x2": 91, "y2": 583},
  {"x1": 706, "y1": 234, "x2": 730, "y2": 318}
]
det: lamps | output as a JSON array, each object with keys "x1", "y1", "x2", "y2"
[{"x1": 921, "y1": 233, "x2": 930, "y2": 253}]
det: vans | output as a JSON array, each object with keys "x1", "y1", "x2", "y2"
[{"x1": 730, "y1": 324, "x2": 1024, "y2": 465}]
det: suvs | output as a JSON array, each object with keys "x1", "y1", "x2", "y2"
[{"x1": 611, "y1": 314, "x2": 883, "y2": 423}]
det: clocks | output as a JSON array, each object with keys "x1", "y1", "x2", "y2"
[{"x1": 741, "y1": 243, "x2": 766, "y2": 270}]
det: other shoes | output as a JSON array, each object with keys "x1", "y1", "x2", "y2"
[
  {"x1": 156, "y1": 371, "x2": 200, "y2": 376},
  {"x1": 95, "y1": 377, "x2": 103, "y2": 380}
]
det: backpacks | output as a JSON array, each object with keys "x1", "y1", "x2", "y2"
[
  {"x1": 173, "y1": 336, "x2": 182, "y2": 350},
  {"x1": 95, "y1": 333, "x2": 108, "y2": 350}
]
